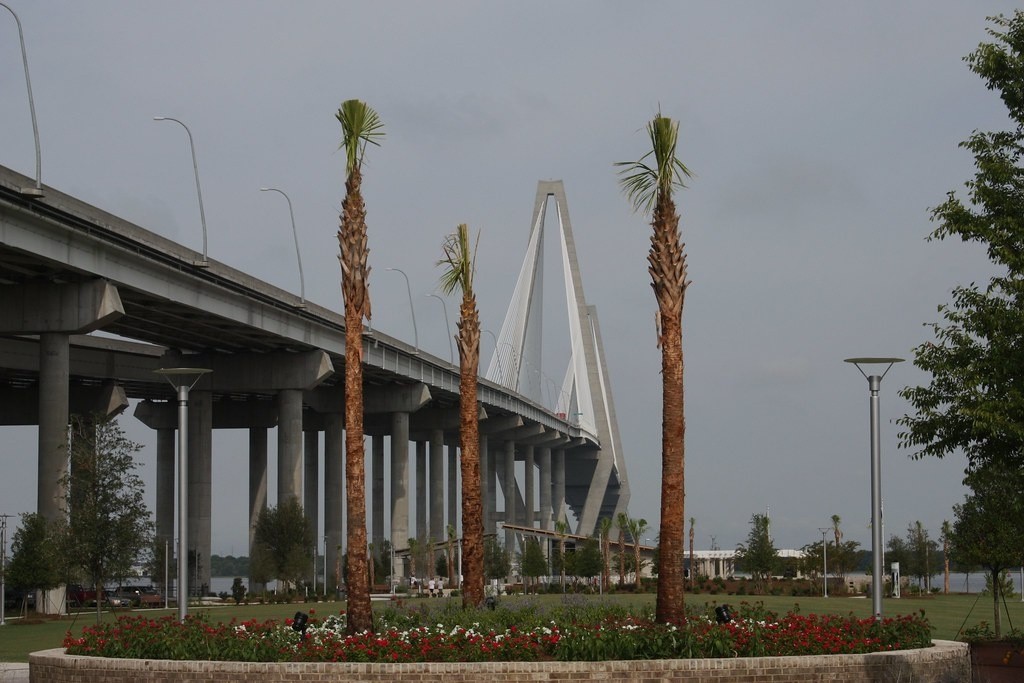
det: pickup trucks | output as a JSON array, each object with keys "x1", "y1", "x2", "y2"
[{"x1": 66, "y1": 584, "x2": 97, "y2": 606}]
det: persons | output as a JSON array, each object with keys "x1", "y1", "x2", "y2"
[
  {"x1": 428, "y1": 577, "x2": 436, "y2": 596},
  {"x1": 436, "y1": 577, "x2": 444, "y2": 593}
]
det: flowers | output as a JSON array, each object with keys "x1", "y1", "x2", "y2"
[{"x1": 960, "y1": 620, "x2": 1024, "y2": 664}]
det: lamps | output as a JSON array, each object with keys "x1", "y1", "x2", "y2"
[
  {"x1": 715, "y1": 607, "x2": 731, "y2": 623},
  {"x1": 293, "y1": 612, "x2": 308, "y2": 631},
  {"x1": 487, "y1": 597, "x2": 495, "y2": 610}
]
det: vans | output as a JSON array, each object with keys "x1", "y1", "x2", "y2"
[{"x1": 115, "y1": 586, "x2": 163, "y2": 607}]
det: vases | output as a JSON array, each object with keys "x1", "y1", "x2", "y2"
[{"x1": 970, "y1": 641, "x2": 1024, "y2": 683}]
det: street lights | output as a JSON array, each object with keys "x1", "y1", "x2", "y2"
[
  {"x1": 320, "y1": 535, "x2": 329, "y2": 595},
  {"x1": 482, "y1": 329, "x2": 504, "y2": 386},
  {"x1": 152, "y1": 368, "x2": 213, "y2": 622},
  {"x1": 386, "y1": 268, "x2": 419, "y2": 351},
  {"x1": 505, "y1": 342, "x2": 572, "y2": 420},
  {"x1": 425, "y1": 294, "x2": 453, "y2": 362},
  {"x1": 845, "y1": 357, "x2": 906, "y2": 620},
  {"x1": 819, "y1": 528, "x2": 831, "y2": 597},
  {"x1": 261, "y1": 187, "x2": 305, "y2": 303},
  {"x1": 0, "y1": 514, "x2": 15, "y2": 624},
  {"x1": 154, "y1": 116, "x2": 207, "y2": 262}
]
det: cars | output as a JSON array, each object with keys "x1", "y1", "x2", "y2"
[{"x1": 97, "y1": 590, "x2": 139, "y2": 608}]
